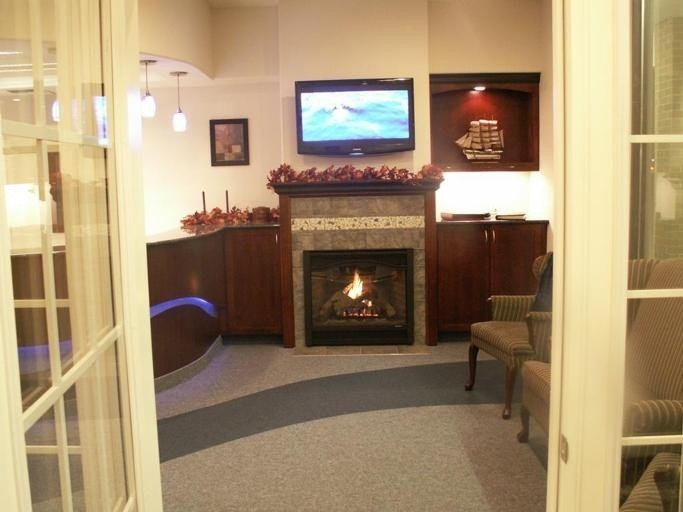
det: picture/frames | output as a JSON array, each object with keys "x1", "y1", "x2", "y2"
[
  {"x1": 431, "y1": 72, "x2": 541, "y2": 171},
  {"x1": 210, "y1": 119, "x2": 249, "y2": 165}
]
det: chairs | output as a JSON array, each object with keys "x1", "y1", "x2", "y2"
[
  {"x1": 465, "y1": 253, "x2": 551, "y2": 418},
  {"x1": 518, "y1": 258, "x2": 683, "y2": 485}
]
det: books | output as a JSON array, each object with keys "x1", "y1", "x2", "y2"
[{"x1": 440, "y1": 213, "x2": 528, "y2": 221}]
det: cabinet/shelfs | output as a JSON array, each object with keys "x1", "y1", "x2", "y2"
[
  {"x1": 438, "y1": 219, "x2": 549, "y2": 332},
  {"x1": 148, "y1": 215, "x2": 281, "y2": 387}
]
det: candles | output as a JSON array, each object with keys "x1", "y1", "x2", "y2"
[
  {"x1": 202, "y1": 190, "x2": 206, "y2": 212},
  {"x1": 226, "y1": 189, "x2": 230, "y2": 212}
]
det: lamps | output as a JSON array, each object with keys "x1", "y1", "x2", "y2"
[
  {"x1": 141, "y1": 59, "x2": 158, "y2": 117},
  {"x1": 170, "y1": 71, "x2": 188, "y2": 133}
]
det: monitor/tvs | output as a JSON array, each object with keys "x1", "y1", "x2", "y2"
[{"x1": 295, "y1": 78, "x2": 415, "y2": 156}]
